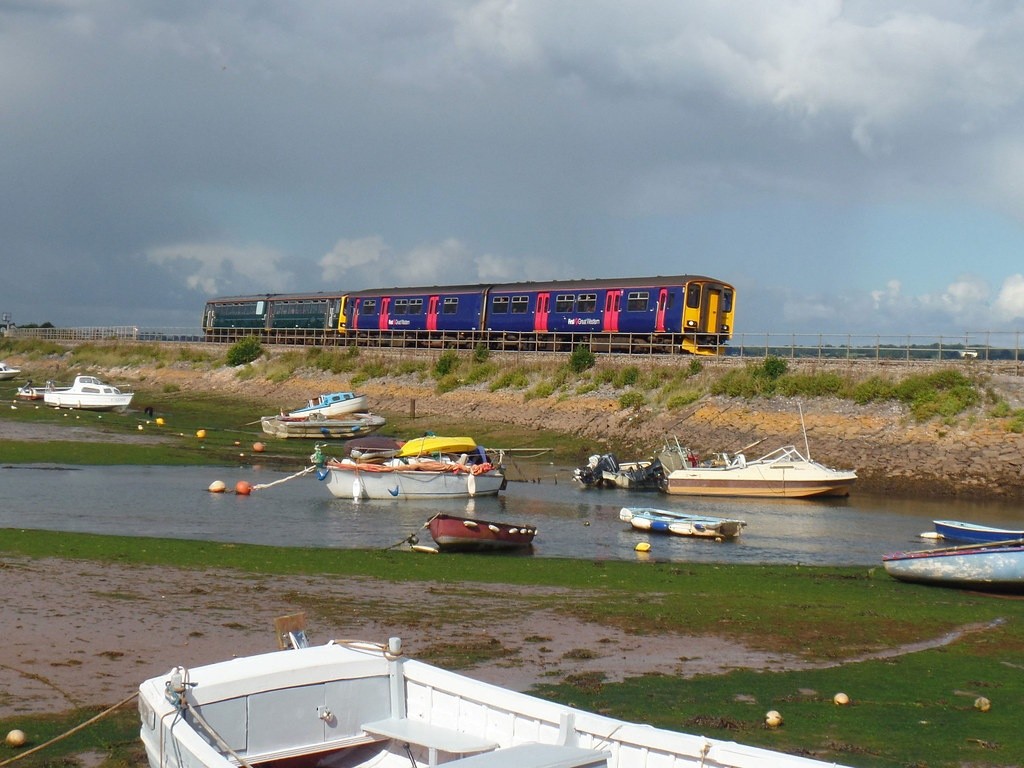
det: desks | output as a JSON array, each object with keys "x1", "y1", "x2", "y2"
[{"x1": 362, "y1": 719, "x2": 498, "y2": 767}]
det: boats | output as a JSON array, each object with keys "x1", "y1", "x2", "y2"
[
  {"x1": 620, "y1": 508, "x2": 747, "y2": 538},
  {"x1": 324, "y1": 444, "x2": 503, "y2": 499},
  {"x1": 15, "y1": 373, "x2": 134, "y2": 408},
  {"x1": 428, "y1": 514, "x2": 536, "y2": 548},
  {"x1": 0, "y1": 363, "x2": 21, "y2": 380},
  {"x1": 261, "y1": 391, "x2": 386, "y2": 439},
  {"x1": 933, "y1": 521, "x2": 1024, "y2": 542},
  {"x1": 666, "y1": 402, "x2": 858, "y2": 498},
  {"x1": 883, "y1": 540, "x2": 1024, "y2": 583},
  {"x1": 138, "y1": 637, "x2": 850, "y2": 768}
]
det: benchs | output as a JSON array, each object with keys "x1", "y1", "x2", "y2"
[
  {"x1": 681, "y1": 518, "x2": 704, "y2": 520},
  {"x1": 426, "y1": 742, "x2": 611, "y2": 768}
]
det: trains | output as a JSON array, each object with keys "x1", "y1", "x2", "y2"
[{"x1": 202, "y1": 274, "x2": 736, "y2": 356}]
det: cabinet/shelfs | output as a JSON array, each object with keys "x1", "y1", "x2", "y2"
[{"x1": 184, "y1": 676, "x2": 391, "y2": 767}]
